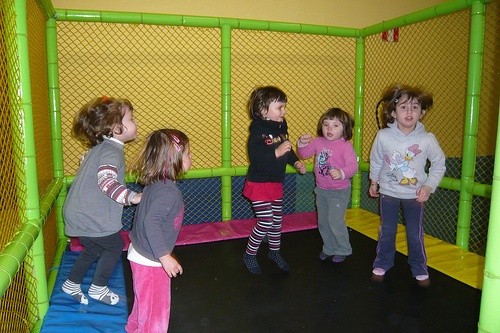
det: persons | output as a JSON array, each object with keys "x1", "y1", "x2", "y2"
[
  {"x1": 61, "y1": 96, "x2": 143, "y2": 305},
  {"x1": 242, "y1": 85, "x2": 308, "y2": 275},
  {"x1": 125, "y1": 129, "x2": 192, "y2": 333},
  {"x1": 296, "y1": 108, "x2": 359, "y2": 262},
  {"x1": 368, "y1": 84, "x2": 446, "y2": 281}
]
